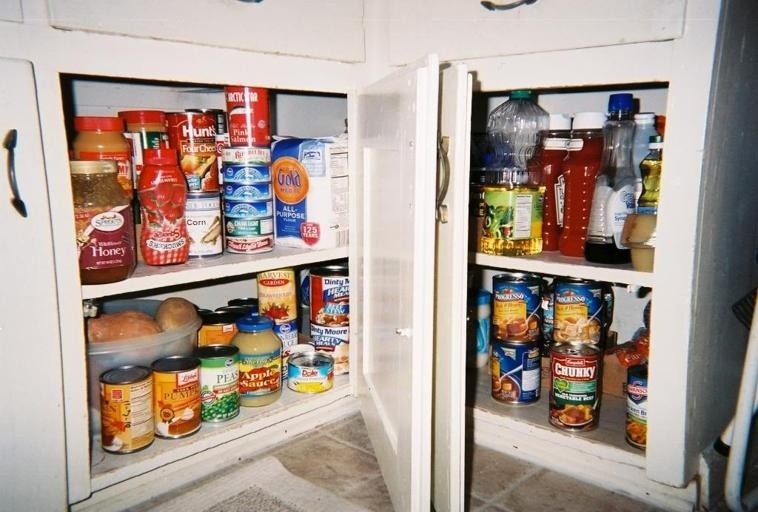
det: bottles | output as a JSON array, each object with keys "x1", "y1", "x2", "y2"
[
  {"x1": 193, "y1": 343, "x2": 241, "y2": 423},
  {"x1": 230, "y1": 314, "x2": 283, "y2": 408},
  {"x1": 185, "y1": 193, "x2": 224, "y2": 260},
  {"x1": 257, "y1": 268, "x2": 298, "y2": 324},
  {"x1": 631, "y1": 113, "x2": 659, "y2": 206},
  {"x1": 526, "y1": 115, "x2": 571, "y2": 252},
  {"x1": 168, "y1": 111, "x2": 219, "y2": 191},
  {"x1": 273, "y1": 322, "x2": 297, "y2": 386},
  {"x1": 559, "y1": 112, "x2": 604, "y2": 257},
  {"x1": 584, "y1": 93, "x2": 637, "y2": 265},
  {"x1": 138, "y1": 148, "x2": 190, "y2": 265},
  {"x1": 272, "y1": 135, "x2": 350, "y2": 252},
  {"x1": 223, "y1": 85, "x2": 271, "y2": 148},
  {"x1": 638, "y1": 135, "x2": 664, "y2": 216},
  {"x1": 198, "y1": 324, "x2": 241, "y2": 347},
  {"x1": 476, "y1": 291, "x2": 491, "y2": 370},
  {"x1": 116, "y1": 110, "x2": 168, "y2": 225},
  {"x1": 73, "y1": 116, "x2": 133, "y2": 199},
  {"x1": 310, "y1": 324, "x2": 349, "y2": 376},
  {"x1": 310, "y1": 276, "x2": 349, "y2": 326},
  {"x1": 222, "y1": 146, "x2": 276, "y2": 254}
]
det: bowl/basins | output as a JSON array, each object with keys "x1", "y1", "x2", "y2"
[{"x1": 79, "y1": 299, "x2": 205, "y2": 441}]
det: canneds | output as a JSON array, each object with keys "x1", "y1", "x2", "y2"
[
  {"x1": 152, "y1": 354, "x2": 202, "y2": 440},
  {"x1": 554, "y1": 279, "x2": 601, "y2": 351},
  {"x1": 287, "y1": 351, "x2": 334, "y2": 394},
  {"x1": 548, "y1": 345, "x2": 598, "y2": 432},
  {"x1": 625, "y1": 365, "x2": 648, "y2": 450},
  {"x1": 491, "y1": 343, "x2": 542, "y2": 405},
  {"x1": 491, "y1": 274, "x2": 544, "y2": 343},
  {"x1": 71, "y1": 159, "x2": 138, "y2": 286},
  {"x1": 99, "y1": 365, "x2": 154, "y2": 454}
]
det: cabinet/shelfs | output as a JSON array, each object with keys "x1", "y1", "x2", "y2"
[{"x1": 1, "y1": 0, "x2": 758, "y2": 512}]
